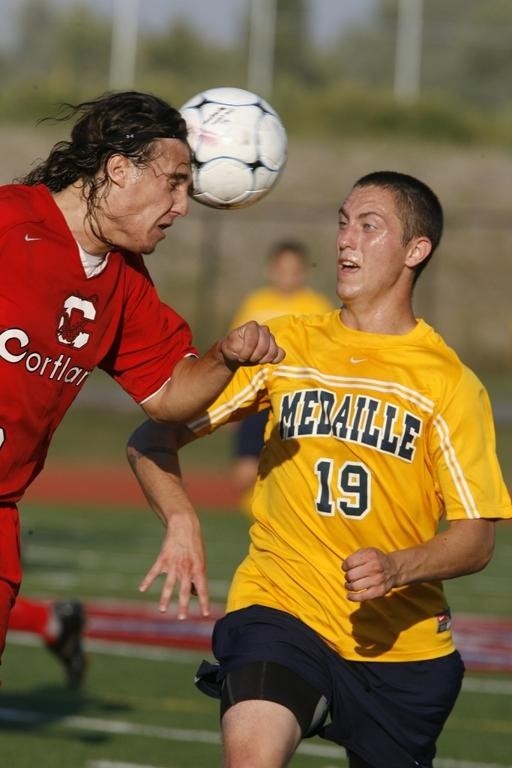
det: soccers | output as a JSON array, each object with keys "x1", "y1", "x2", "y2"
[{"x1": 179, "y1": 86, "x2": 290, "y2": 208}]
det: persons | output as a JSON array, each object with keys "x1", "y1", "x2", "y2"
[
  {"x1": 124, "y1": 169, "x2": 510, "y2": 767},
  {"x1": 7, "y1": 595, "x2": 92, "y2": 691},
  {"x1": 223, "y1": 238, "x2": 337, "y2": 508},
  {"x1": 0, "y1": 91, "x2": 287, "y2": 693}
]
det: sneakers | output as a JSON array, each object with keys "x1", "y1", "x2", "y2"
[{"x1": 46, "y1": 600, "x2": 86, "y2": 691}]
